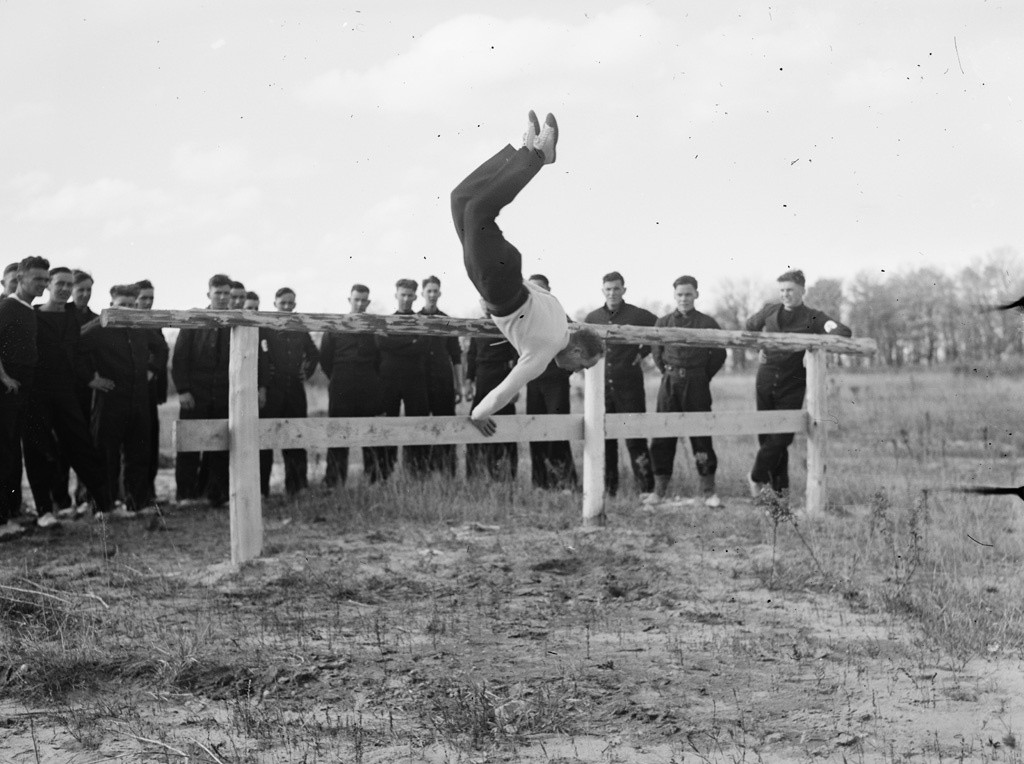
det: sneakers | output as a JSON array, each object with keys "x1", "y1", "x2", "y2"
[
  {"x1": 534, "y1": 113, "x2": 558, "y2": 163},
  {"x1": 523, "y1": 110, "x2": 541, "y2": 152}
]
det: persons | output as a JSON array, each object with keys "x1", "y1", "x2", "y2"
[
  {"x1": 581, "y1": 271, "x2": 658, "y2": 500},
  {"x1": 641, "y1": 276, "x2": 727, "y2": 509},
  {"x1": 746, "y1": 270, "x2": 852, "y2": 526},
  {"x1": 0, "y1": 255, "x2": 464, "y2": 540},
  {"x1": 526, "y1": 275, "x2": 579, "y2": 497},
  {"x1": 450, "y1": 111, "x2": 603, "y2": 438},
  {"x1": 465, "y1": 299, "x2": 520, "y2": 487}
]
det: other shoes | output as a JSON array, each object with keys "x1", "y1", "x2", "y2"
[
  {"x1": 59, "y1": 500, "x2": 163, "y2": 518},
  {"x1": 642, "y1": 492, "x2": 667, "y2": 505},
  {"x1": 37, "y1": 511, "x2": 61, "y2": 529},
  {"x1": 700, "y1": 492, "x2": 721, "y2": 507},
  {"x1": 0, "y1": 520, "x2": 26, "y2": 538},
  {"x1": 637, "y1": 492, "x2": 649, "y2": 504},
  {"x1": 746, "y1": 469, "x2": 762, "y2": 505},
  {"x1": 168, "y1": 497, "x2": 229, "y2": 508}
]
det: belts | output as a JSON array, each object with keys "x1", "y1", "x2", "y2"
[
  {"x1": 485, "y1": 287, "x2": 523, "y2": 312},
  {"x1": 664, "y1": 366, "x2": 707, "y2": 378}
]
